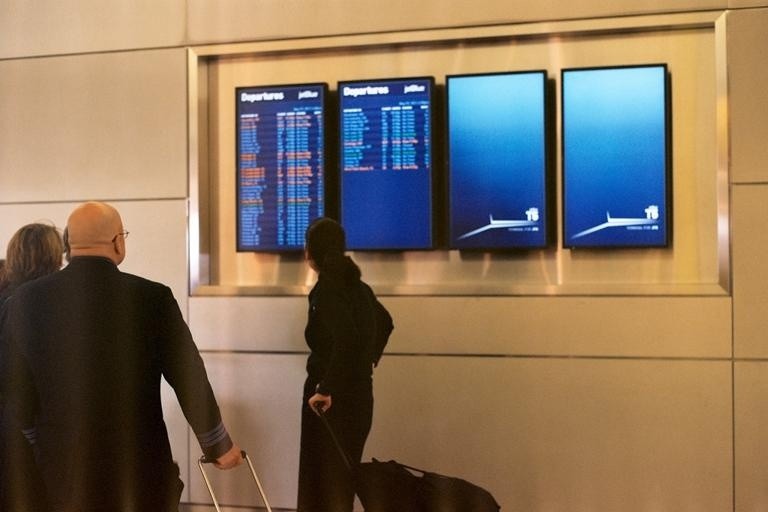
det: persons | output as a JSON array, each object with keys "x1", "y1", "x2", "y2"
[
  {"x1": 0, "y1": 200, "x2": 243, "y2": 512},
  {"x1": 293, "y1": 214, "x2": 396, "y2": 510},
  {"x1": 0, "y1": 220, "x2": 64, "y2": 303}
]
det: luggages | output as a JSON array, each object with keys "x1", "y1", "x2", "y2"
[{"x1": 317, "y1": 401, "x2": 501, "y2": 512}]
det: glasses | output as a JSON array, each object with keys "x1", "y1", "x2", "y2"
[{"x1": 112, "y1": 229, "x2": 130, "y2": 243}]
